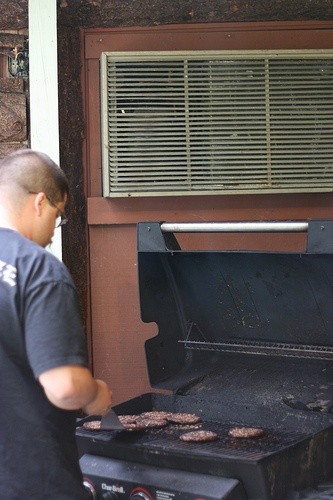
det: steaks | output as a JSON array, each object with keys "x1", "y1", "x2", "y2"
[{"x1": 83, "y1": 410, "x2": 263, "y2": 443}]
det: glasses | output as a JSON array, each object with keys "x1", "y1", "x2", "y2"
[{"x1": 28, "y1": 190, "x2": 70, "y2": 228}]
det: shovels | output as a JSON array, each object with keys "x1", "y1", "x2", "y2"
[{"x1": 99, "y1": 392, "x2": 126, "y2": 432}]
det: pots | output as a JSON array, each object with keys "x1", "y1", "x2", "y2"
[{"x1": 74, "y1": 391, "x2": 333, "y2": 500}]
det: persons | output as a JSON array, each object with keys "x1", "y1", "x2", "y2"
[{"x1": 0, "y1": 146, "x2": 114, "y2": 500}]
